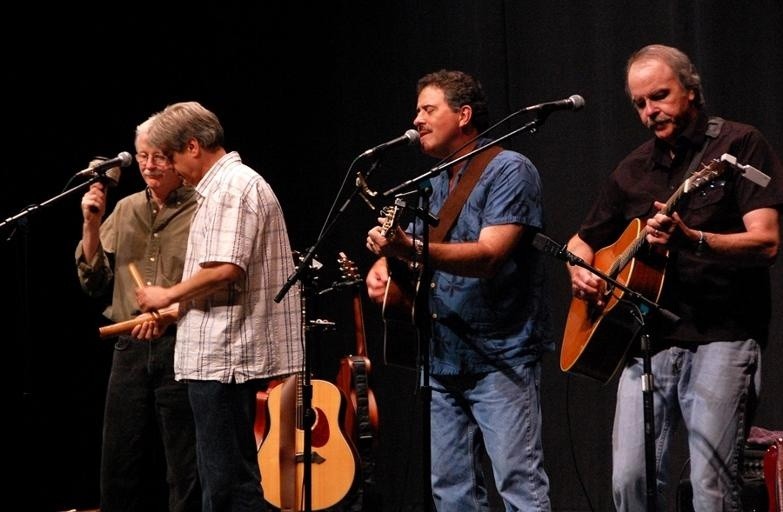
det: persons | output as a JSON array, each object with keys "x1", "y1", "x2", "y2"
[
  {"x1": 365, "y1": 71, "x2": 561, "y2": 512},
  {"x1": 72, "y1": 113, "x2": 199, "y2": 508},
  {"x1": 129, "y1": 102, "x2": 305, "y2": 511},
  {"x1": 564, "y1": 44, "x2": 782, "y2": 510}
]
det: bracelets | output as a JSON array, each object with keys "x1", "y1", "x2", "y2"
[
  {"x1": 412, "y1": 238, "x2": 424, "y2": 258},
  {"x1": 697, "y1": 229, "x2": 705, "y2": 254}
]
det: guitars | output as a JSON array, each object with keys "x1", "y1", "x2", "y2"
[
  {"x1": 559, "y1": 152, "x2": 771, "y2": 383},
  {"x1": 381, "y1": 199, "x2": 422, "y2": 371},
  {"x1": 257, "y1": 252, "x2": 378, "y2": 512}
]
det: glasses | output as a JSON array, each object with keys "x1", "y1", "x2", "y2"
[{"x1": 135, "y1": 152, "x2": 169, "y2": 166}]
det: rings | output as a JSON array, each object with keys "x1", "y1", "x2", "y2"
[
  {"x1": 369, "y1": 242, "x2": 375, "y2": 247},
  {"x1": 654, "y1": 227, "x2": 659, "y2": 236},
  {"x1": 577, "y1": 288, "x2": 585, "y2": 296}
]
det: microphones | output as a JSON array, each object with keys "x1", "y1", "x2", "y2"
[
  {"x1": 81, "y1": 150, "x2": 132, "y2": 174},
  {"x1": 525, "y1": 94, "x2": 587, "y2": 109},
  {"x1": 532, "y1": 233, "x2": 584, "y2": 267},
  {"x1": 365, "y1": 129, "x2": 419, "y2": 154}
]
